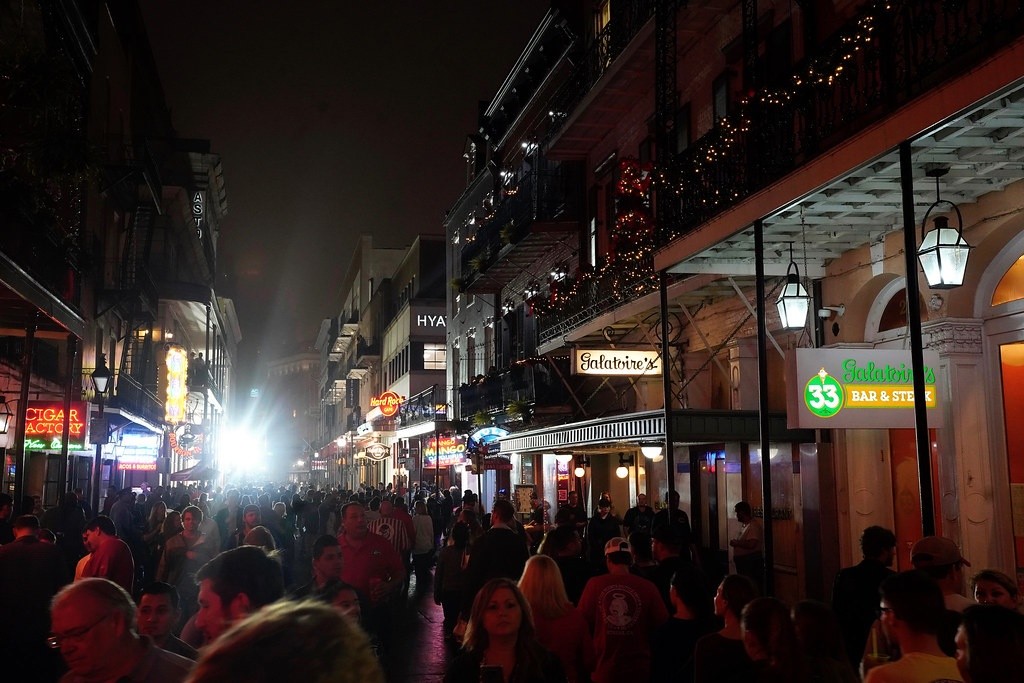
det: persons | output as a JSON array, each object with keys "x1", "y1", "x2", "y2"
[
  {"x1": 576, "y1": 536, "x2": 669, "y2": 683},
  {"x1": 729, "y1": 502, "x2": 764, "y2": 582},
  {"x1": 832, "y1": 526, "x2": 900, "y2": 665},
  {"x1": 649, "y1": 564, "x2": 858, "y2": 683},
  {"x1": 336, "y1": 501, "x2": 406, "y2": 633},
  {"x1": 971, "y1": 570, "x2": 1018, "y2": 607},
  {"x1": 186, "y1": 598, "x2": 382, "y2": 683},
  {"x1": 136, "y1": 581, "x2": 199, "y2": 661},
  {"x1": 954, "y1": 603, "x2": 1024, "y2": 683},
  {"x1": 434, "y1": 522, "x2": 472, "y2": 638},
  {"x1": 195, "y1": 545, "x2": 284, "y2": 644},
  {"x1": 441, "y1": 578, "x2": 570, "y2": 683},
  {"x1": 472, "y1": 500, "x2": 529, "y2": 580},
  {"x1": 863, "y1": 573, "x2": 964, "y2": 683},
  {"x1": 0, "y1": 482, "x2": 690, "y2": 624},
  {"x1": 49, "y1": 578, "x2": 197, "y2": 683},
  {"x1": 516, "y1": 555, "x2": 596, "y2": 683},
  {"x1": 910, "y1": 535, "x2": 974, "y2": 611}
]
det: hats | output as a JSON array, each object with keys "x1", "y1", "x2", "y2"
[
  {"x1": 604, "y1": 537, "x2": 631, "y2": 556},
  {"x1": 910, "y1": 536, "x2": 971, "y2": 567}
]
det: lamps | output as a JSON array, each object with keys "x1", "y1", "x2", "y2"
[
  {"x1": 554, "y1": 451, "x2": 574, "y2": 463},
  {"x1": 640, "y1": 443, "x2": 665, "y2": 458},
  {"x1": 915, "y1": 162, "x2": 977, "y2": 289},
  {"x1": 616, "y1": 452, "x2": 634, "y2": 479},
  {"x1": 574, "y1": 456, "x2": 590, "y2": 478},
  {"x1": 774, "y1": 241, "x2": 814, "y2": 331}
]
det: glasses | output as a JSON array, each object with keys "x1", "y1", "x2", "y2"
[
  {"x1": 875, "y1": 607, "x2": 892, "y2": 618},
  {"x1": 48, "y1": 615, "x2": 106, "y2": 648}
]
